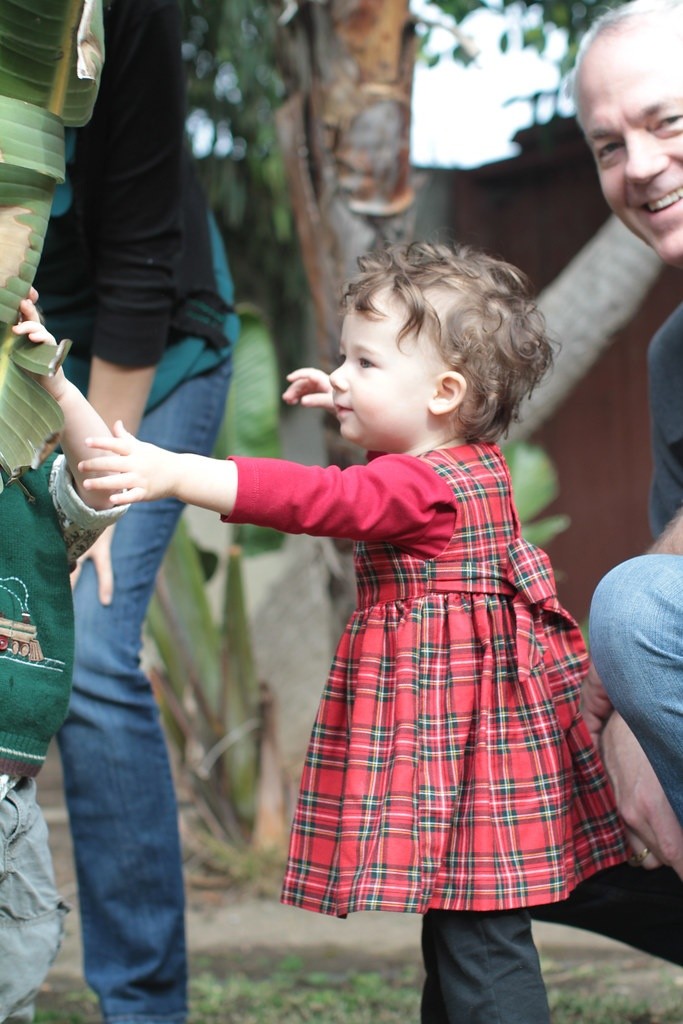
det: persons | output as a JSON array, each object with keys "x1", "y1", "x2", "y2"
[
  {"x1": 78, "y1": 243, "x2": 683, "y2": 1024},
  {"x1": 0, "y1": 0, "x2": 241, "y2": 1023},
  {"x1": 573, "y1": 0, "x2": 683, "y2": 882}
]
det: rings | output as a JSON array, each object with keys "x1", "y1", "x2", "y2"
[{"x1": 631, "y1": 848, "x2": 650, "y2": 865}]
